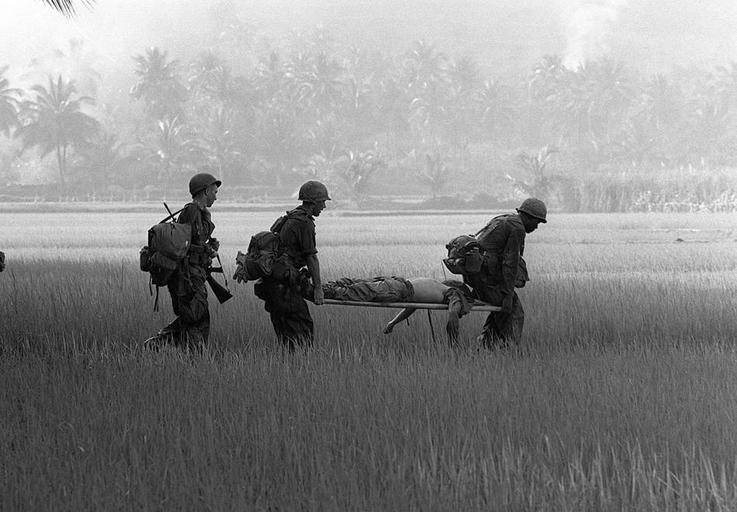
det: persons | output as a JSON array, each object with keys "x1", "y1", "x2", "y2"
[
  {"x1": 469, "y1": 198, "x2": 547, "y2": 353},
  {"x1": 145, "y1": 173, "x2": 221, "y2": 360},
  {"x1": 255, "y1": 181, "x2": 332, "y2": 353},
  {"x1": 298, "y1": 268, "x2": 474, "y2": 350}
]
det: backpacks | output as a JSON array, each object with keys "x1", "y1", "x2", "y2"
[
  {"x1": 140, "y1": 222, "x2": 192, "y2": 311},
  {"x1": 442, "y1": 222, "x2": 501, "y2": 275},
  {"x1": 232, "y1": 230, "x2": 280, "y2": 284}
]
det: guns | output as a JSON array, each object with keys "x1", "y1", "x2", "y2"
[{"x1": 163, "y1": 202, "x2": 233, "y2": 305}]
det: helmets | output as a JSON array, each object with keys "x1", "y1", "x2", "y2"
[
  {"x1": 298, "y1": 181, "x2": 331, "y2": 202},
  {"x1": 518, "y1": 198, "x2": 547, "y2": 223},
  {"x1": 190, "y1": 173, "x2": 222, "y2": 196}
]
